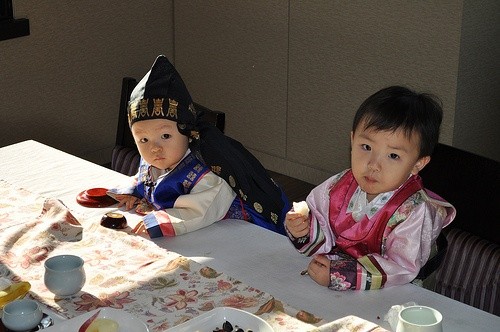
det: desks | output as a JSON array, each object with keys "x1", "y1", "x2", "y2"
[{"x1": 0, "y1": 140, "x2": 500, "y2": 332}]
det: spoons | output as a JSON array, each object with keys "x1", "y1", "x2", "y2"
[{"x1": 37, "y1": 315, "x2": 54, "y2": 331}]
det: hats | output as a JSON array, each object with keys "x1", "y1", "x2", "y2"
[{"x1": 127, "y1": 53, "x2": 200, "y2": 135}]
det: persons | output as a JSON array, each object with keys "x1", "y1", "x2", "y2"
[
  {"x1": 118, "y1": 55, "x2": 290, "y2": 239},
  {"x1": 284, "y1": 86, "x2": 457, "y2": 291}
]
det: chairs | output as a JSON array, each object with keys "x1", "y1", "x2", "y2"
[
  {"x1": 410, "y1": 141, "x2": 500, "y2": 319},
  {"x1": 111, "y1": 77, "x2": 226, "y2": 177}
]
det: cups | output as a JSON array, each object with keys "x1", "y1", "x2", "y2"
[
  {"x1": 44, "y1": 253, "x2": 86, "y2": 297},
  {"x1": 395, "y1": 306, "x2": 444, "y2": 332},
  {"x1": 1, "y1": 299, "x2": 43, "y2": 332}
]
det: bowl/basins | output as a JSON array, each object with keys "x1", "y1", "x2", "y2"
[
  {"x1": 101, "y1": 212, "x2": 128, "y2": 229},
  {"x1": 162, "y1": 307, "x2": 274, "y2": 332},
  {"x1": 36, "y1": 306, "x2": 151, "y2": 332}
]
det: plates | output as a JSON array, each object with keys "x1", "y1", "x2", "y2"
[{"x1": 76, "y1": 187, "x2": 117, "y2": 208}]
